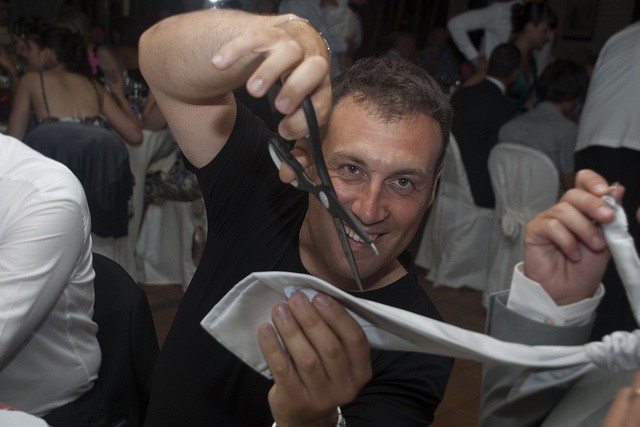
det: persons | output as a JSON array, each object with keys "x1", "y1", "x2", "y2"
[
  {"x1": 478, "y1": 169, "x2": 640, "y2": 427},
  {"x1": 510, "y1": 1, "x2": 558, "y2": 110},
  {"x1": 137, "y1": 7, "x2": 454, "y2": 427},
  {"x1": 420, "y1": 28, "x2": 459, "y2": 86},
  {"x1": 496, "y1": 59, "x2": 590, "y2": 199},
  {"x1": 523, "y1": 36, "x2": 590, "y2": 124},
  {"x1": 0, "y1": 33, "x2": 29, "y2": 105},
  {"x1": 371, "y1": 29, "x2": 419, "y2": 71},
  {"x1": 267, "y1": 0, "x2": 363, "y2": 80},
  {"x1": 131, "y1": 89, "x2": 213, "y2": 347},
  {"x1": 573, "y1": 21, "x2": 640, "y2": 341},
  {"x1": 0, "y1": 19, "x2": 143, "y2": 145},
  {"x1": 0, "y1": 134, "x2": 107, "y2": 427},
  {"x1": 443, "y1": 43, "x2": 466, "y2": 75},
  {"x1": 447, "y1": 0, "x2": 554, "y2": 111}
]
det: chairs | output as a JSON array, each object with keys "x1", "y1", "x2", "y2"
[
  {"x1": 481, "y1": 142, "x2": 560, "y2": 309},
  {"x1": 410, "y1": 131, "x2": 495, "y2": 288}
]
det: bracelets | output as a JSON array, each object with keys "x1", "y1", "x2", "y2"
[
  {"x1": 270, "y1": 405, "x2": 351, "y2": 427},
  {"x1": 287, "y1": 18, "x2": 332, "y2": 60}
]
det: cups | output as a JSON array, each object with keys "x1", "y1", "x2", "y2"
[{"x1": 128, "y1": 82, "x2": 144, "y2": 106}]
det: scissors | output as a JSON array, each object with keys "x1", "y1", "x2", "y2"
[{"x1": 267, "y1": 95, "x2": 379, "y2": 293}]
